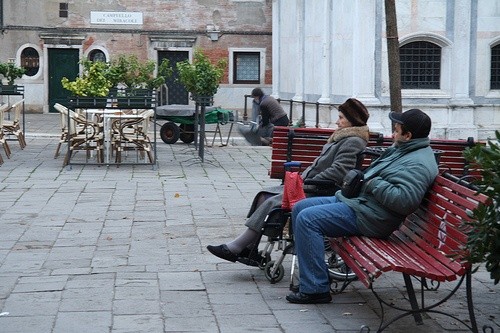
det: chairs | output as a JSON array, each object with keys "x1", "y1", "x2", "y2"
[
  {"x1": 53, "y1": 102, "x2": 157, "y2": 166},
  {"x1": 0, "y1": 98, "x2": 27, "y2": 166}
]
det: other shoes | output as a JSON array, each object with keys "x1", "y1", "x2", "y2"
[
  {"x1": 285, "y1": 292, "x2": 332, "y2": 303},
  {"x1": 238, "y1": 246, "x2": 258, "y2": 260},
  {"x1": 290, "y1": 284, "x2": 299, "y2": 293},
  {"x1": 207, "y1": 243, "x2": 237, "y2": 262}
]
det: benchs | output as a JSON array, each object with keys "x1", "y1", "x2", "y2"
[{"x1": 268, "y1": 125, "x2": 495, "y2": 333}]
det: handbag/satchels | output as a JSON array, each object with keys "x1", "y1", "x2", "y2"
[{"x1": 341, "y1": 168, "x2": 364, "y2": 198}]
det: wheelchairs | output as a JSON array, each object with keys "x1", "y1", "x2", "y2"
[{"x1": 235, "y1": 148, "x2": 383, "y2": 284}]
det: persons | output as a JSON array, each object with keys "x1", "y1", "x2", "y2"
[
  {"x1": 206, "y1": 98, "x2": 369, "y2": 264},
  {"x1": 286, "y1": 109, "x2": 439, "y2": 304},
  {"x1": 251, "y1": 88, "x2": 290, "y2": 128}
]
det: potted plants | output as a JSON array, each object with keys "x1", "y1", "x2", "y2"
[
  {"x1": 62, "y1": 54, "x2": 174, "y2": 106},
  {"x1": 176, "y1": 49, "x2": 228, "y2": 106},
  {"x1": 0, "y1": 61, "x2": 27, "y2": 94}
]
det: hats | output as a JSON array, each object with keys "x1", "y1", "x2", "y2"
[
  {"x1": 338, "y1": 97, "x2": 370, "y2": 126},
  {"x1": 389, "y1": 108, "x2": 431, "y2": 134}
]
